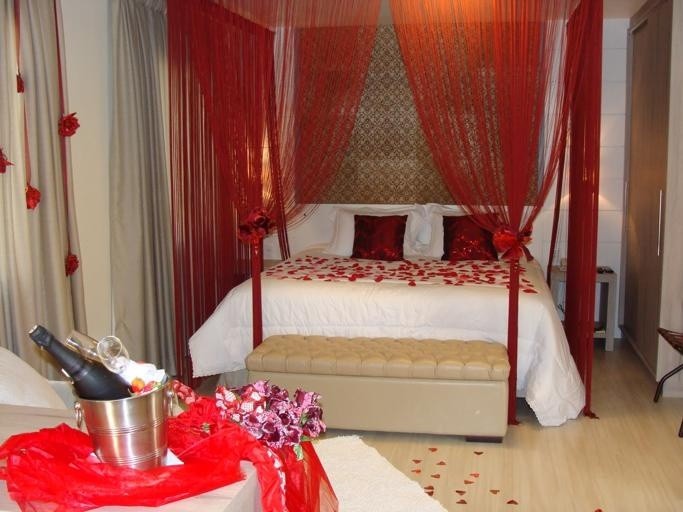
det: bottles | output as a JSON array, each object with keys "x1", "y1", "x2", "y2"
[{"x1": 26, "y1": 325, "x2": 130, "y2": 401}]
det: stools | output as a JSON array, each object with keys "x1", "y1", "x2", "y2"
[{"x1": 651, "y1": 325, "x2": 683, "y2": 440}]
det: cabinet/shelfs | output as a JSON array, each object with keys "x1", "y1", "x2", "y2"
[{"x1": 617, "y1": 3, "x2": 672, "y2": 384}]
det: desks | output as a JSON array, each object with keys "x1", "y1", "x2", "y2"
[
  {"x1": 0, "y1": 406, "x2": 291, "y2": 512},
  {"x1": 548, "y1": 263, "x2": 617, "y2": 352}
]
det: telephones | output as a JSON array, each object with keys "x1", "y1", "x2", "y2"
[{"x1": 560, "y1": 258, "x2": 567, "y2": 272}]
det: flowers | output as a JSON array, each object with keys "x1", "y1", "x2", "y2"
[{"x1": 217, "y1": 380, "x2": 329, "y2": 464}]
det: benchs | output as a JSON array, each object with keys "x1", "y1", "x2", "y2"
[{"x1": 241, "y1": 331, "x2": 512, "y2": 444}]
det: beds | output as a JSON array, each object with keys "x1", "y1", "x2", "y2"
[{"x1": 185, "y1": 201, "x2": 592, "y2": 426}]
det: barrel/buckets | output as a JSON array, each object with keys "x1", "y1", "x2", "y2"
[{"x1": 70, "y1": 371, "x2": 171, "y2": 472}]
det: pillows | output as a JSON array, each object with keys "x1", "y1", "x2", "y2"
[
  {"x1": 324, "y1": 206, "x2": 423, "y2": 255},
  {"x1": 441, "y1": 212, "x2": 501, "y2": 262},
  {"x1": 349, "y1": 213, "x2": 408, "y2": 261},
  {"x1": 424, "y1": 202, "x2": 516, "y2": 258}
]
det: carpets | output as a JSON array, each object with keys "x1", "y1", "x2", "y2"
[{"x1": 307, "y1": 433, "x2": 449, "y2": 511}]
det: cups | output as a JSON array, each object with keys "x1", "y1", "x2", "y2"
[
  {"x1": 62, "y1": 329, "x2": 102, "y2": 362},
  {"x1": 96, "y1": 335, "x2": 129, "y2": 375}
]
